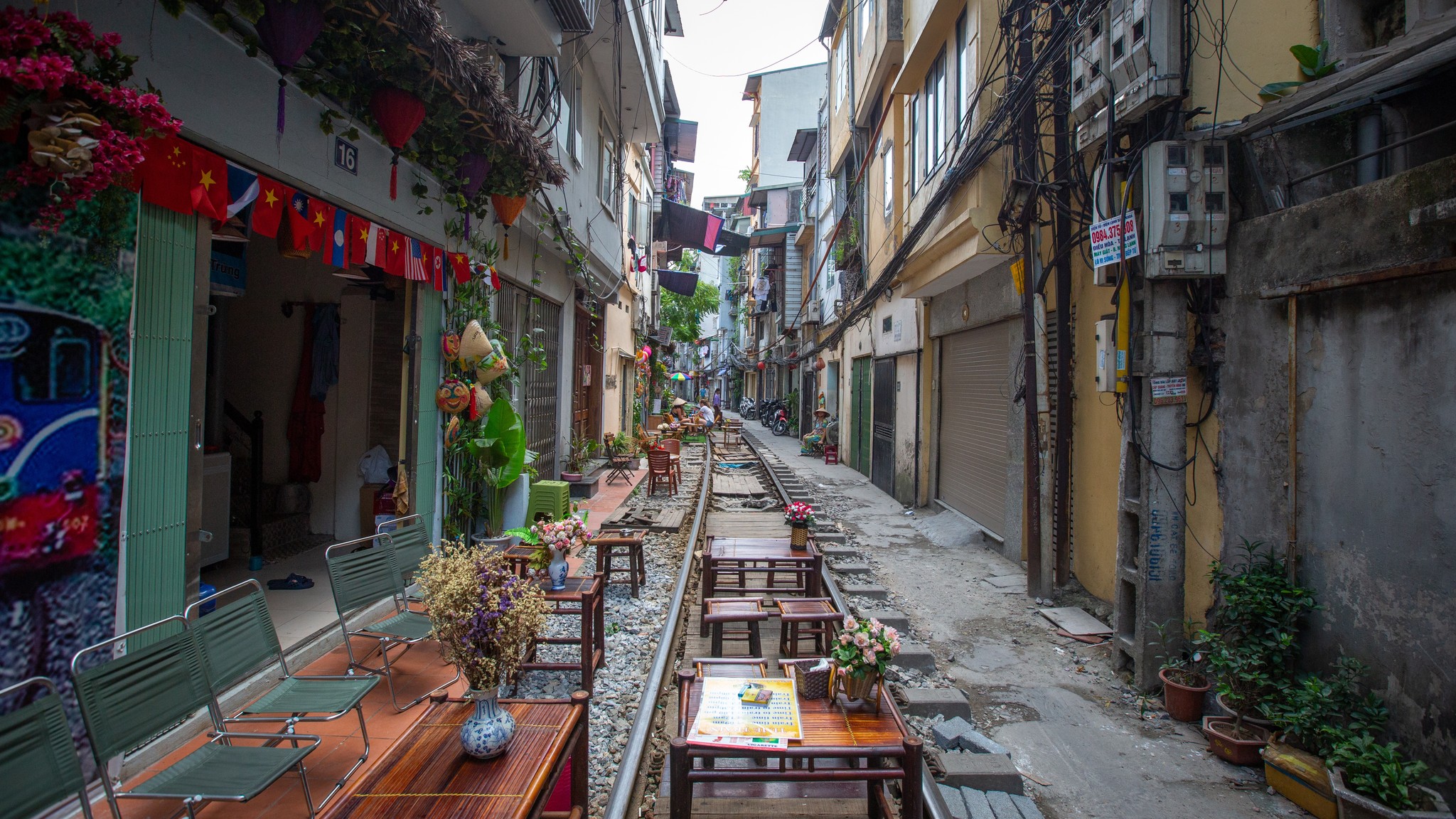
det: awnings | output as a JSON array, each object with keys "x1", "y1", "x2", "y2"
[
  {"x1": 664, "y1": 117, "x2": 698, "y2": 163},
  {"x1": 664, "y1": 60, "x2": 680, "y2": 119},
  {"x1": 695, "y1": 337, "x2": 709, "y2": 343},
  {"x1": 787, "y1": 128, "x2": 817, "y2": 161},
  {"x1": 664, "y1": 0, "x2": 684, "y2": 37},
  {"x1": 652, "y1": 269, "x2": 699, "y2": 297},
  {"x1": 742, "y1": 73, "x2": 761, "y2": 100},
  {"x1": 748, "y1": 225, "x2": 801, "y2": 249},
  {"x1": 645, "y1": 335, "x2": 665, "y2": 348},
  {"x1": 667, "y1": 243, "x2": 683, "y2": 262},
  {"x1": 747, "y1": 311, "x2": 771, "y2": 317},
  {"x1": 672, "y1": 168, "x2": 694, "y2": 206},
  {"x1": 725, "y1": 208, "x2": 737, "y2": 216},
  {"x1": 717, "y1": 369, "x2": 726, "y2": 376}
]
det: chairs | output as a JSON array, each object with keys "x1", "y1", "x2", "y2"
[
  {"x1": 0, "y1": 512, "x2": 447, "y2": 819},
  {"x1": 600, "y1": 400, "x2": 746, "y2": 499},
  {"x1": 811, "y1": 436, "x2": 826, "y2": 459}
]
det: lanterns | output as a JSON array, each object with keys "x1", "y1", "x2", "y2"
[
  {"x1": 758, "y1": 361, "x2": 765, "y2": 370},
  {"x1": 811, "y1": 358, "x2": 825, "y2": 388},
  {"x1": 368, "y1": 88, "x2": 427, "y2": 202},
  {"x1": 635, "y1": 346, "x2": 652, "y2": 364},
  {"x1": 455, "y1": 151, "x2": 490, "y2": 239},
  {"x1": 492, "y1": 189, "x2": 527, "y2": 261}
]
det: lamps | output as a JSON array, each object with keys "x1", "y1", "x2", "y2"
[{"x1": 329, "y1": 250, "x2": 371, "y2": 281}]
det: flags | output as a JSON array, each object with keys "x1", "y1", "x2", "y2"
[{"x1": 145, "y1": 135, "x2": 502, "y2": 290}]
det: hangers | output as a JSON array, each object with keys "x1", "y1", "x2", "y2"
[{"x1": 699, "y1": 276, "x2": 765, "y2": 347}]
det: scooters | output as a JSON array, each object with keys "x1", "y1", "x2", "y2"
[{"x1": 738, "y1": 396, "x2": 790, "y2": 436}]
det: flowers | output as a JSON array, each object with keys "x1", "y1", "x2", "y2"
[
  {"x1": 780, "y1": 500, "x2": 820, "y2": 530},
  {"x1": 503, "y1": 414, "x2": 701, "y2": 579},
  {"x1": 413, "y1": 532, "x2": 552, "y2": 698},
  {"x1": 723, "y1": 418, "x2": 731, "y2": 423},
  {"x1": 831, "y1": 615, "x2": 903, "y2": 676}
]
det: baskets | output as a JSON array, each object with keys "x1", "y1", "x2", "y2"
[
  {"x1": 793, "y1": 658, "x2": 831, "y2": 701},
  {"x1": 840, "y1": 664, "x2": 880, "y2": 699},
  {"x1": 790, "y1": 522, "x2": 809, "y2": 550}
]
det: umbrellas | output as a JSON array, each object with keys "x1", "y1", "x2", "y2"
[{"x1": 668, "y1": 372, "x2": 694, "y2": 400}]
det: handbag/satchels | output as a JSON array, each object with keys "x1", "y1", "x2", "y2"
[{"x1": 358, "y1": 444, "x2": 395, "y2": 484}]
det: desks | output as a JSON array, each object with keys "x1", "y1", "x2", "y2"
[
  {"x1": 700, "y1": 533, "x2": 824, "y2": 637},
  {"x1": 589, "y1": 528, "x2": 650, "y2": 599},
  {"x1": 672, "y1": 668, "x2": 924, "y2": 819},
  {"x1": 720, "y1": 426, "x2": 742, "y2": 448},
  {"x1": 316, "y1": 568, "x2": 607, "y2": 819},
  {"x1": 645, "y1": 453, "x2": 682, "y2": 484},
  {"x1": 683, "y1": 422, "x2": 704, "y2": 441}
]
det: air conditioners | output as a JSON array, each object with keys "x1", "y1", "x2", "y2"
[
  {"x1": 732, "y1": 300, "x2": 820, "y2": 360},
  {"x1": 682, "y1": 362, "x2": 685, "y2": 367},
  {"x1": 652, "y1": 270, "x2": 660, "y2": 294}
]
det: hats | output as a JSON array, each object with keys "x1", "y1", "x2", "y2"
[
  {"x1": 477, "y1": 338, "x2": 509, "y2": 386},
  {"x1": 813, "y1": 407, "x2": 830, "y2": 418},
  {"x1": 471, "y1": 381, "x2": 494, "y2": 423},
  {"x1": 679, "y1": 398, "x2": 688, "y2": 404},
  {"x1": 459, "y1": 319, "x2": 493, "y2": 372},
  {"x1": 671, "y1": 397, "x2": 685, "y2": 405}
]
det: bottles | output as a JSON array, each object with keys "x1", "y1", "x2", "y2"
[{"x1": 686, "y1": 412, "x2": 688, "y2": 418}]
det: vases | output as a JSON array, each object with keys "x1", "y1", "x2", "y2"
[
  {"x1": 661, "y1": 429, "x2": 667, "y2": 433},
  {"x1": 457, "y1": 681, "x2": 518, "y2": 759},
  {"x1": 695, "y1": 420, "x2": 699, "y2": 424},
  {"x1": 672, "y1": 428, "x2": 678, "y2": 431},
  {"x1": 547, "y1": 544, "x2": 569, "y2": 591},
  {"x1": 725, "y1": 423, "x2": 728, "y2": 427}
]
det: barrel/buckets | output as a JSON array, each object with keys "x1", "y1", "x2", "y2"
[
  {"x1": 249, "y1": 556, "x2": 262, "y2": 571},
  {"x1": 199, "y1": 582, "x2": 217, "y2": 618}
]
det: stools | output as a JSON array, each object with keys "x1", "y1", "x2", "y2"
[
  {"x1": 502, "y1": 478, "x2": 572, "y2": 579},
  {"x1": 700, "y1": 563, "x2": 843, "y2": 687},
  {"x1": 824, "y1": 445, "x2": 838, "y2": 465}
]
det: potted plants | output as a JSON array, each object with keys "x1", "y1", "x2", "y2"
[
  {"x1": 1146, "y1": 535, "x2": 1456, "y2": 819},
  {"x1": 466, "y1": 395, "x2": 540, "y2": 555},
  {"x1": 561, "y1": 424, "x2": 603, "y2": 481},
  {"x1": 782, "y1": 388, "x2": 799, "y2": 437}
]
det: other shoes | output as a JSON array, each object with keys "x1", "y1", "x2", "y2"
[
  {"x1": 798, "y1": 452, "x2": 813, "y2": 457},
  {"x1": 690, "y1": 434, "x2": 698, "y2": 437},
  {"x1": 715, "y1": 424, "x2": 719, "y2": 426}
]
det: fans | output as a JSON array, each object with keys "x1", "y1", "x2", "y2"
[{"x1": 826, "y1": 421, "x2": 839, "y2": 445}]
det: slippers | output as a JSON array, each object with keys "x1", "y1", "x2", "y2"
[{"x1": 267, "y1": 573, "x2": 315, "y2": 590}]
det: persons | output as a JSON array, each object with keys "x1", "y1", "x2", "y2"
[
  {"x1": 670, "y1": 397, "x2": 698, "y2": 436},
  {"x1": 712, "y1": 388, "x2": 721, "y2": 426},
  {"x1": 699, "y1": 385, "x2": 709, "y2": 401},
  {"x1": 691, "y1": 399, "x2": 715, "y2": 434},
  {"x1": 798, "y1": 407, "x2": 830, "y2": 456},
  {"x1": 693, "y1": 386, "x2": 698, "y2": 405}
]
